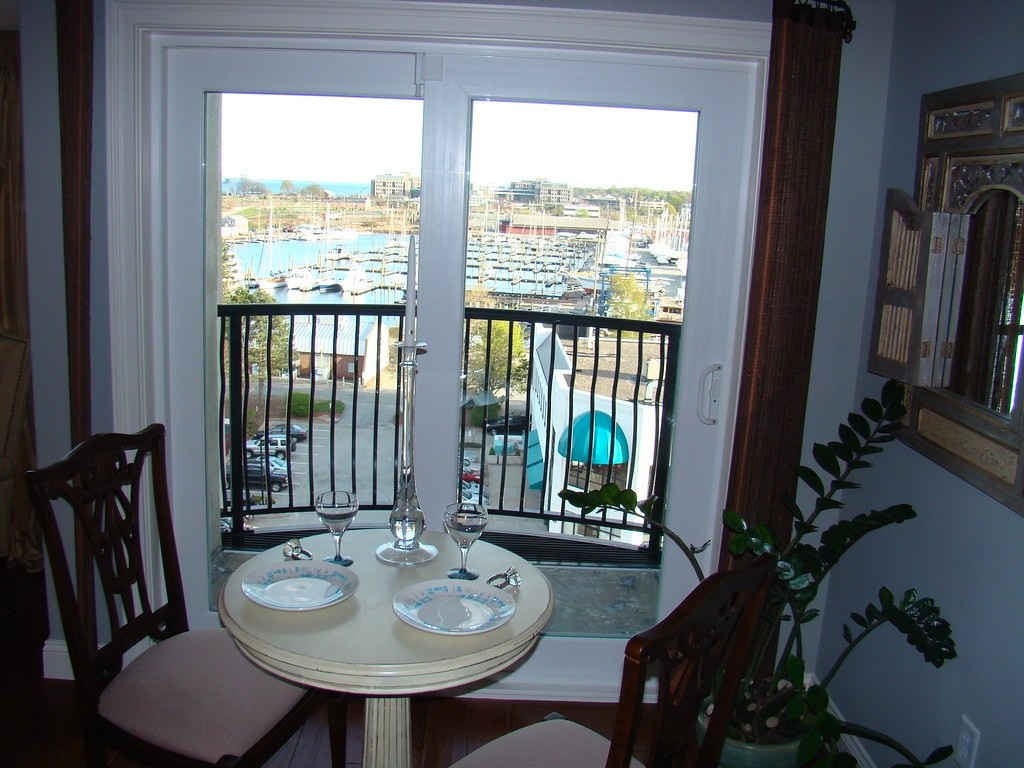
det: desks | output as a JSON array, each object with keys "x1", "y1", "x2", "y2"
[{"x1": 215, "y1": 532, "x2": 554, "y2": 768}]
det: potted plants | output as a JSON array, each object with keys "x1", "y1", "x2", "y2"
[{"x1": 559, "y1": 370, "x2": 959, "y2": 768}]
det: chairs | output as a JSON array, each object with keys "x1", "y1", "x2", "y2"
[
  {"x1": 442, "y1": 543, "x2": 780, "y2": 768},
  {"x1": 24, "y1": 419, "x2": 352, "y2": 768}
]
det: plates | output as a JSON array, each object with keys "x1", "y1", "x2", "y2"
[
  {"x1": 241, "y1": 560, "x2": 360, "y2": 612},
  {"x1": 393, "y1": 579, "x2": 516, "y2": 635}
]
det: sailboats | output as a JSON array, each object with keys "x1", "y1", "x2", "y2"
[{"x1": 242, "y1": 197, "x2": 691, "y2": 307}]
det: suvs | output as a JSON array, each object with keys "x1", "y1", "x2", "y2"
[{"x1": 246, "y1": 434, "x2": 297, "y2": 460}]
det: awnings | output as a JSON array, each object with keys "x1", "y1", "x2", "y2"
[
  {"x1": 522, "y1": 429, "x2": 543, "y2": 489},
  {"x1": 558, "y1": 410, "x2": 629, "y2": 465}
]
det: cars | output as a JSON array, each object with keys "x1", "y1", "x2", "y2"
[
  {"x1": 459, "y1": 450, "x2": 478, "y2": 463},
  {"x1": 247, "y1": 456, "x2": 293, "y2": 472},
  {"x1": 226, "y1": 462, "x2": 288, "y2": 492},
  {"x1": 458, "y1": 457, "x2": 481, "y2": 471},
  {"x1": 458, "y1": 463, "x2": 481, "y2": 484},
  {"x1": 456, "y1": 488, "x2": 489, "y2": 505},
  {"x1": 253, "y1": 422, "x2": 307, "y2": 441},
  {"x1": 457, "y1": 478, "x2": 489, "y2": 499},
  {"x1": 486, "y1": 413, "x2": 526, "y2": 436}
]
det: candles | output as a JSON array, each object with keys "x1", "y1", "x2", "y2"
[{"x1": 404, "y1": 230, "x2": 417, "y2": 347}]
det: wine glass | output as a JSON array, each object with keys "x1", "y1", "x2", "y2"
[
  {"x1": 442, "y1": 503, "x2": 488, "y2": 579},
  {"x1": 315, "y1": 490, "x2": 359, "y2": 567}
]
